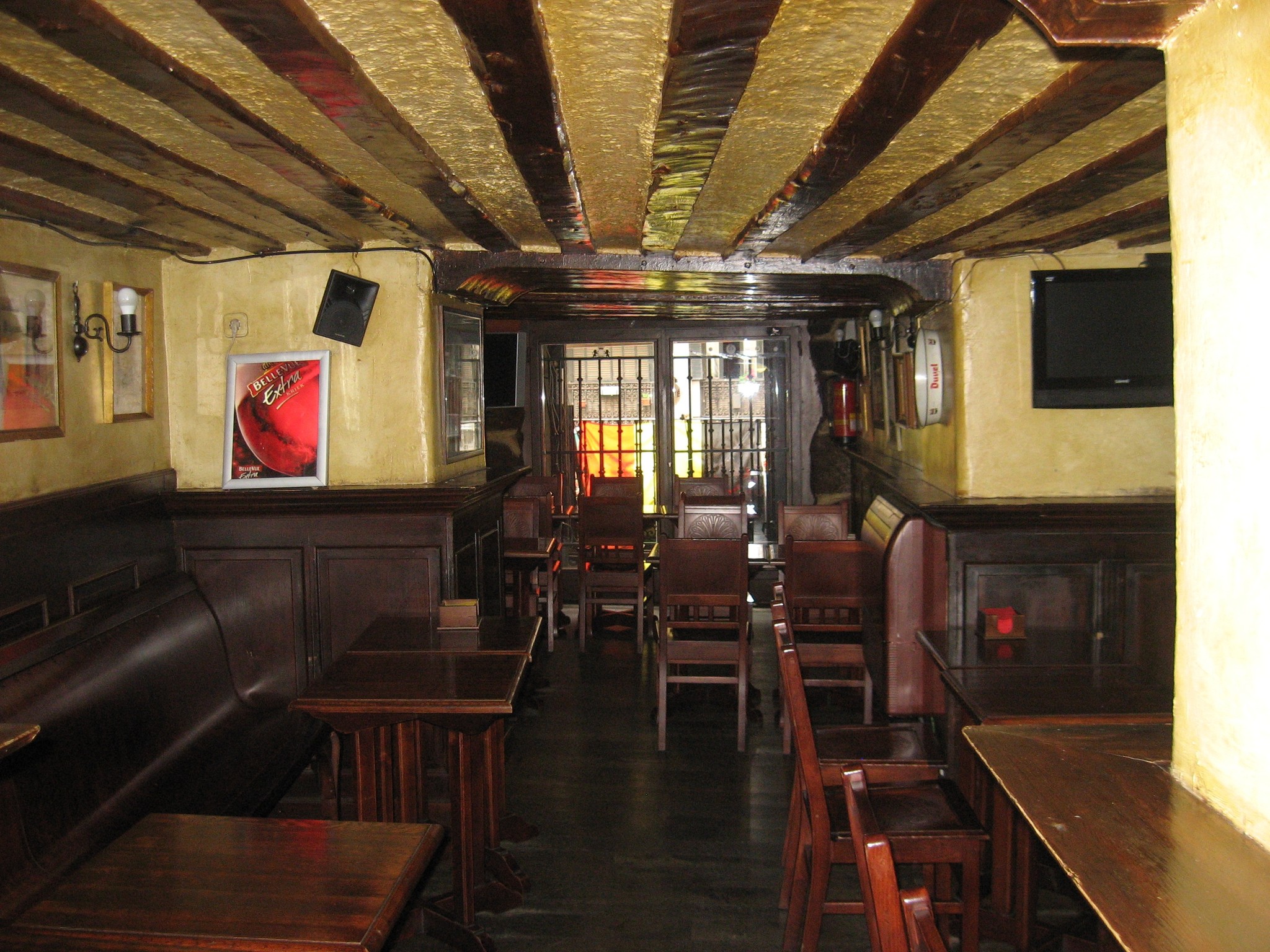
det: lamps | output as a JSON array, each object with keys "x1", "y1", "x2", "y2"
[
  {"x1": 19, "y1": 288, "x2": 54, "y2": 355},
  {"x1": 72, "y1": 279, "x2": 142, "y2": 363},
  {"x1": 834, "y1": 308, "x2": 919, "y2": 375}
]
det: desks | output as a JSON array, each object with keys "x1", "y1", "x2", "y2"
[
  {"x1": 961, "y1": 721, "x2": 1270, "y2": 952},
  {"x1": 914, "y1": 627, "x2": 1174, "y2": 903},
  {"x1": 503, "y1": 511, "x2": 861, "y2": 636},
  {"x1": 0, "y1": 813, "x2": 444, "y2": 952},
  {"x1": 285, "y1": 614, "x2": 545, "y2": 952}
]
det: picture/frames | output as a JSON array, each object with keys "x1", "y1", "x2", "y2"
[
  {"x1": 222, "y1": 350, "x2": 331, "y2": 490},
  {"x1": 103, "y1": 280, "x2": 156, "y2": 425},
  {"x1": 438, "y1": 304, "x2": 486, "y2": 465},
  {"x1": 0, "y1": 260, "x2": 66, "y2": 443}
]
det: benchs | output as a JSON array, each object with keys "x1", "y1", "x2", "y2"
[{"x1": 0, "y1": 564, "x2": 329, "y2": 924}]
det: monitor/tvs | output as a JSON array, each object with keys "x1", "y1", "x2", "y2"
[
  {"x1": 483, "y1": 331, "x2": 526, "y2": 408},
  {"x1": 1030, "y1": 267, "x2": 1173, "y2": 409}
]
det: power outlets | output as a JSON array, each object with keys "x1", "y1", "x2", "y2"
[{"x1": 223, "y1": 312, "x2": 248, "y2": 337}]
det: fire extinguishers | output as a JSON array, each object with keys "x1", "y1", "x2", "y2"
[{"x1": 826, "y1": 366, "x2": 858, "y2": 437}]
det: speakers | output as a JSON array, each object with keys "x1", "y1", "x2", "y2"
[{"x1": 312, "y1": 269, "x2": 380, "y2": 347}]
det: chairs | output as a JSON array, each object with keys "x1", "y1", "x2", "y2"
[
  {"x1": 771, "y1": 497, "x2": 991, "y2": 952},
  {"x1": 503, "y1": 468, "x2": 749, "y2": 756}
]
